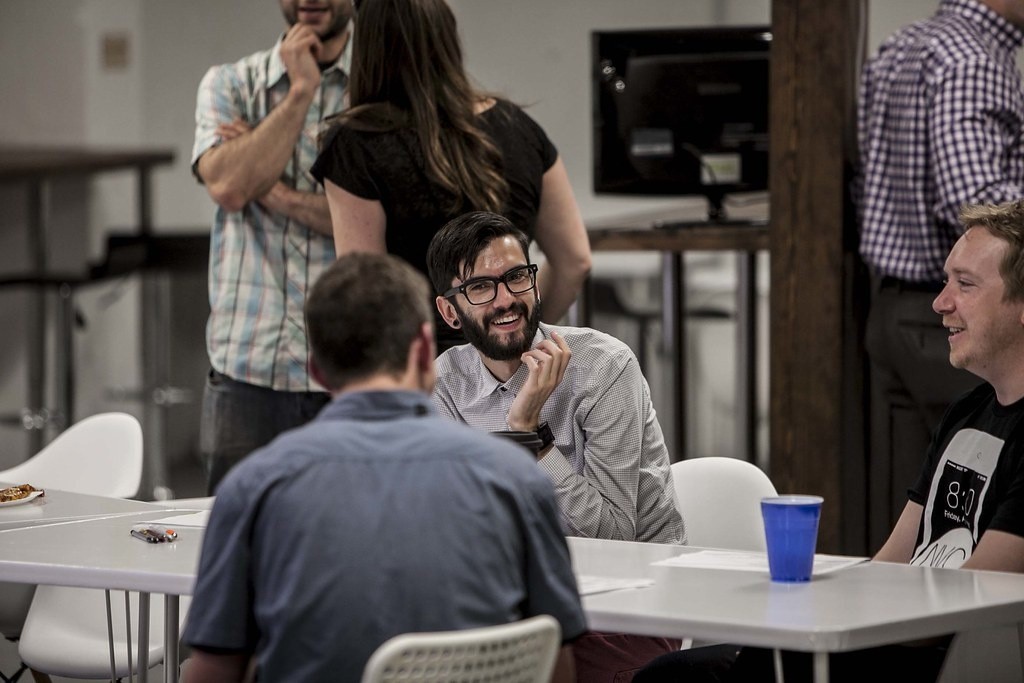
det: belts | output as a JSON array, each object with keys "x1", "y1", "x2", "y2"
[{"x1": 883, "y1": 277, "x2": 944, "y2": 293}]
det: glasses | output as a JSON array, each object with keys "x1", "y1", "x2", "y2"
[{"x1": 443, "y1": 264, "x2": 538, "y2": 305}]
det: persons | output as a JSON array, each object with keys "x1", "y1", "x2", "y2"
[
  {"x1": 309, "y1": 0, "x2": 592, "y2": 380},
  {"x1": 426, "y1": 210, "x2": 691, "y2": 682},
  {"x1": 179, "y1": 249, "x2": 586, "y2": 683},
  {"x1": 849, "y1": 0, "x2": 1024, "y2": 565},
  {"x1": 189, "y1": 0, "x2": 416, "y2": 498},
  {"x1": 723, "y1": 193, "x2": 1024, "y2": 683}
]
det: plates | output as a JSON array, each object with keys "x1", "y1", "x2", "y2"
[{"x1": 0, "y1": 488, "x2": 43, "y2": 508}]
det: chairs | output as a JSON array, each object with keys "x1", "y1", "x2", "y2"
[
  {"x1": 0, "y1": 413, "x2": 217, "y2": 682},
  {"x1": 668, "y1": 457, "x2": 780, "y2": 649},
  {"x1": 364, "y1": 617, "x2": 562, "y2": 682}
]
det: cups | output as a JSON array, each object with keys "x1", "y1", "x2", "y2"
[
  {"x1": 488, "y1": 429, "x2": 542, "y2": 462},
  {"x1": 759, "y1": 494, "x2": 824, "y2": 584}
]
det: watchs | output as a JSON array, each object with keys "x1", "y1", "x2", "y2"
[{"x1": 529, "y1": 421, "x2": 553, "y2": 457}]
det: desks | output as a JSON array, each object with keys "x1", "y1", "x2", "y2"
[{"x1": 0, "y1": 487, "x2": 1024, "y2": 683}]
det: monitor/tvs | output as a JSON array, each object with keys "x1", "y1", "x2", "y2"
[{"x1": 591, "y1": 23, "x2": 772, "y2": 229}]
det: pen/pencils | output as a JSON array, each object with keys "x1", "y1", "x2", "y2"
[{"x1": 130, "y1": 524, "x2": 178, "y2": 543}]
